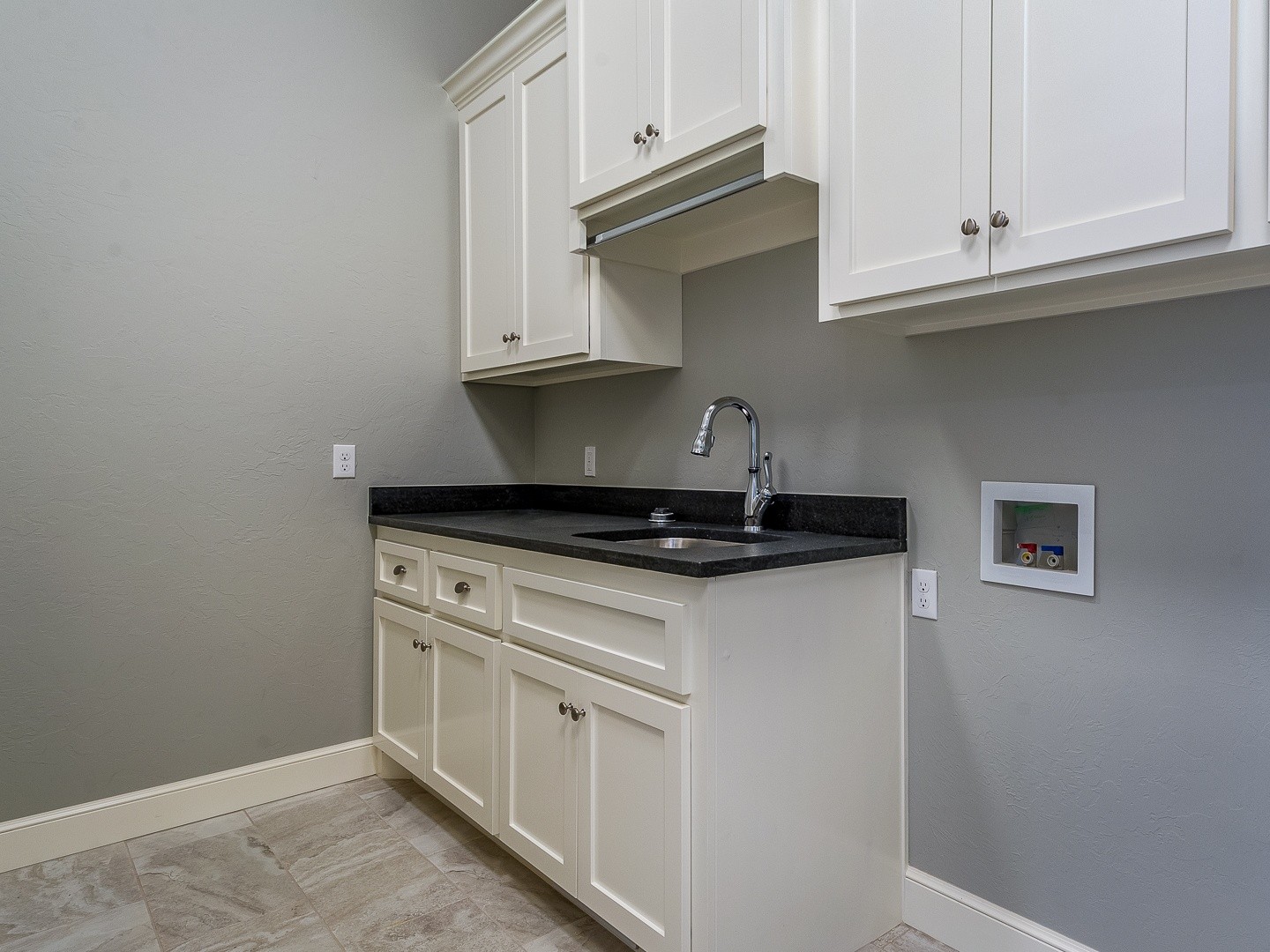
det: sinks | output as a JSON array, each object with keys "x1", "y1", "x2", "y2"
[{"x1": 571, "y1": 528, "x2": 792, "y2": 549}]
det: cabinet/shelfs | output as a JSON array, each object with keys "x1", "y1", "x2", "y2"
[
  {"x1": 455, "y1": 0, "x2": 1270, "y2": 387},
  {"x1": 373, "y1": 537, "x2": 902, "y2": 951}
]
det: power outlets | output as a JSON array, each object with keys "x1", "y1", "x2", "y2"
[
  {"x1": 584, "y1": 446, "x2": 596, "y2": 477},
  {"x1": 332, "y1": 444, "x2": 356, "y2": 479},
  {"x1": 911, "y1": 568, "x2": 938, "y2": 620}
]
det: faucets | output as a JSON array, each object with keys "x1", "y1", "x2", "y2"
[{"x1": 690, "y1": 397, "x2": 778, "y2": 532}]
